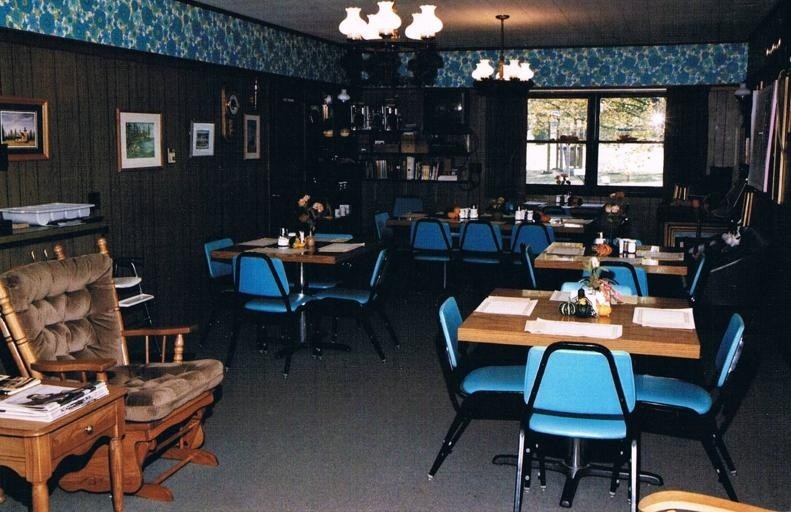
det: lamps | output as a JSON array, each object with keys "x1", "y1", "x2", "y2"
[
  {"x1": 337, "y1": 0, "x2": 443, "y2": 84},
  {"x1": 471, "y1": 11, "x2": 535, "y2": 89}
]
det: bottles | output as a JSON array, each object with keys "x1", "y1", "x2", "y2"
[
  {"x1": 459, "y1": 208, "x2": 478, "y2": 219},
  {"x1": 514, "y1": 209, "x2": 534, "y2": 221},
  {"x1": 558, "y1": 287, "x2": 611, "y2": 317},
  {"x1": 395, "y1": 164, "x2": 400, "y2": 176},
  {"x1": 560, "y1": 191, "x2": 572, "y2": 205},
  {"x1": 616, "y1": 238, "x2": 637, "y2": 254},
  {"x1": 278, "y1": 228, "x2": 305, "y2": 247}
]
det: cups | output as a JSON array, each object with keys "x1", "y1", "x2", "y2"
[
  {"x1": 421, "y1": 166, "x2": 430, "y2": 181},
  {"x1": 693, "y1": 198, "x2": 702, "y2": 209},
  {"x1": 334, "y1": 204, "x2": 351, "y2": 218},
  {"x1": 594, "y1": 238, "x2": 606, "y2": 254}
]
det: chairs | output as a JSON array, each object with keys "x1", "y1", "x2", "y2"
[
  {"x1": 0, "y1": 239, "x2": 224, "y2": 502},
  {"x1": 205, "y1": 231, "x2": 400, "y2": 366}
]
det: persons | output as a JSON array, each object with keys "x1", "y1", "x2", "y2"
[{"x1": 25, "y1": 387, "x2": 80, "y2": 407}]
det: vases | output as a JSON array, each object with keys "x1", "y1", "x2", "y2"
[{"x1": 306, "y1": 224, "x2": 315, "y2": 247}]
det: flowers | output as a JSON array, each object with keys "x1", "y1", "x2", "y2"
[{"x1": 297, "y1": 192, "x2": 322, "y2": 224}]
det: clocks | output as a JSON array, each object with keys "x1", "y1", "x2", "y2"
[{"x1": 221, "y1": 82, "x2": 243, "y2": 142}]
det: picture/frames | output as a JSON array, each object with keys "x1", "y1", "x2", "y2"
[
  {"x1": 0, "y1": 95, "x2": 52, "y2": 162},
  {"x1": 116, "y1": 104, "x2": 261, "y2": 173}
]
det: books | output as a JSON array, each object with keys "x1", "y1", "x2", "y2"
[
  {"x1": 362, "y1": 157, "x2": 460, "y2": 182},
  {"x1": 0, "y1": 375, "x2": 110, "y2": 423}
]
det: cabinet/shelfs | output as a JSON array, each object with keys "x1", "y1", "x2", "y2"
[{"x1": 2, "y1": 372, "x2": 126, "y2": 512}]
